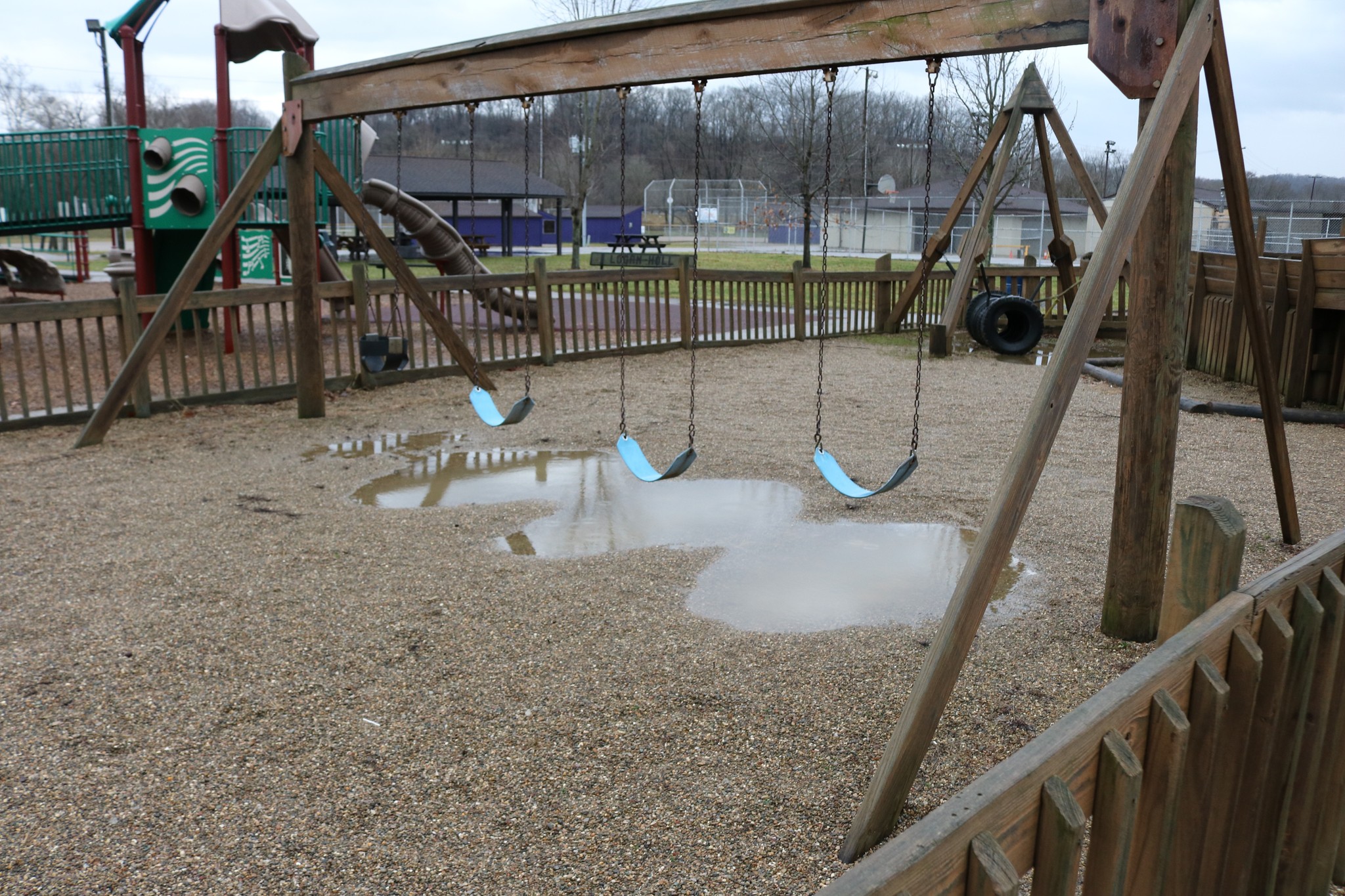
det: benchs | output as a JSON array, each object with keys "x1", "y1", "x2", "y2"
[
  {"x1": 468, "y1": 243, "x2": 490, "y2": 257},
  {"x1": 608, "y1": 243, "x2": 666, "y2": 254},
  {"x1": 369, "y1": 263, "x2": 436, "y2": 279}
]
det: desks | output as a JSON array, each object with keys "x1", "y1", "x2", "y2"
[
  {"x1": 613, "y1": 233, "x2": 664, "y2": 255},
  {"x1": 462, "y1": 235, "x2": 496, "y2": 257}
]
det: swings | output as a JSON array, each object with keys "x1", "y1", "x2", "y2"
[
  {"x1": 813, "y1": 53, "x2": 942, "y2": 500},
  {"x1": 464, "y1": 95, "x2": 534, "y2": 427},
  {"x1": 348, "y1": 108, "x2": 409, "y2": 374},
  {"x1": 615, "y1": 77, "x2": 709, "y2": 482}
]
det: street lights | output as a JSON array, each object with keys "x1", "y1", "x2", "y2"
[
  {"x1": 854, "y1": 67, "x2": 878, "y2": 214},
  {"x1": 85, "y1": 18, "x2": 125, "y2": 252},
  {"x1": 897, "y1": 143, "x2": 928, "y2": 188}
]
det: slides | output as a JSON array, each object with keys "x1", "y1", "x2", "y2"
[{"x1": 360, "y1": 178, "x2": 554, "y2": 328}]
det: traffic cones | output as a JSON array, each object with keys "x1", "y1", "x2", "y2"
[
  {"x1": 1043, "y1": 250, "x2": 1047, "y2": 259},
  {"x1": 1009, "y1": 250, "x2": 1012, "y2": 258}
]
